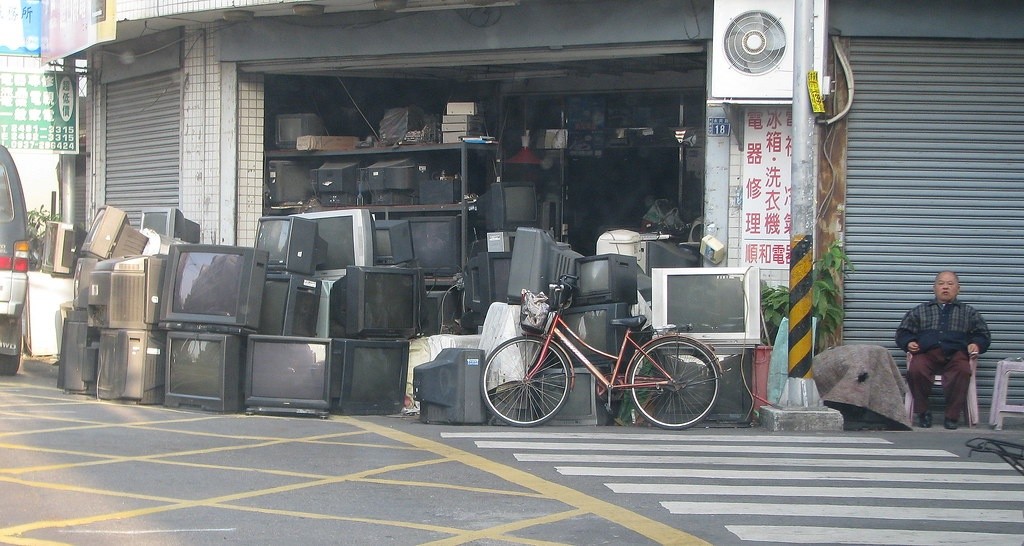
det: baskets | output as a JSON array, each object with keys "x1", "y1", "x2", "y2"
[{"x1": 520, "y1": 291, "x2": 549, "y2": 333}]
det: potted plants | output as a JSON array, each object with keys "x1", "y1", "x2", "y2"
[{"x1": 752, "y1": 240, "x2": 854, "y2": 420}]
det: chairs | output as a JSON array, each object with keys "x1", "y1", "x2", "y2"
[{"x1": 904, "y1": 352, "x2": 979, "y2": 428}]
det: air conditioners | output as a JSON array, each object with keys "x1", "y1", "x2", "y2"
[{"x1": 707, "y1": 0, "x2": 833, "y2": 108}]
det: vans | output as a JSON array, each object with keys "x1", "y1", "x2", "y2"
[{"x1": 0, "y1": 145, "x2": 29, "y2": 375}]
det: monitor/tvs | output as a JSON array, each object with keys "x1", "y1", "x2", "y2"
[{"x1": 24, "y1": 113, "x2": 761, "y2": 423}]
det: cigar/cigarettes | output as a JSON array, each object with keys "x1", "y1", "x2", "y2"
[{"x1": 972, "y1": 351, "x2": 978, "y2": 354}]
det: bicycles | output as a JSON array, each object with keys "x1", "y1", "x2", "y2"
[{"x1": 480, "y1": 274, "x2": 723, "y2": 431}]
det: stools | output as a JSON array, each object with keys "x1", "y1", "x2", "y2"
[{"x1": 989, "y1": 360, "x2": 1024, "y2": 430}]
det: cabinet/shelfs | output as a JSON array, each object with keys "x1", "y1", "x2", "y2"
[{"x1": 261, "y1": 141, "x2": 502, "y2": 335}]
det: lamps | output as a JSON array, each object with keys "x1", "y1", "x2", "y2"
[
  {"x1": 292, "y1": 4, "x2": 325, "y2": 16},
  {"x1": 373, "y1": 0, "x2": 407, "y2": 12},
  {"x1": 222, "y1": 11, "x2": 254, "y2": 22},
  {"x1": 505, "y1": 97, "x2": 545, "y2": 165}
]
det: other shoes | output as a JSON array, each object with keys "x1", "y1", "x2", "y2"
[
  {"x1": 919, "y1": 410, "x2": 932, "y2": 428},
  {"x1": 945, "y1": 417, "x2": 958, "y2": 429}
]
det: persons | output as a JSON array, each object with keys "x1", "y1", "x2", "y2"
[{"x1": 895, "y1": 271, "x2": 991, "y2": 429}]
